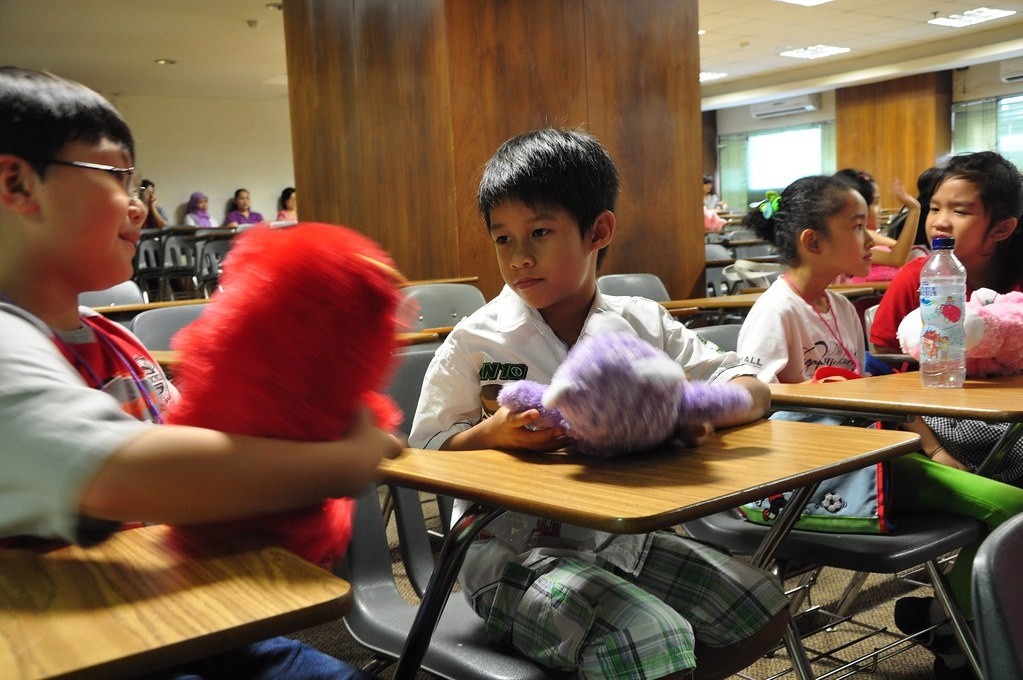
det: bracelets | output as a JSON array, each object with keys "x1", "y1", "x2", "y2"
[{"x1": 928, "y1": 446, "x2": 943, "y2": 458}]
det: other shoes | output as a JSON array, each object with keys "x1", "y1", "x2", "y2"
[{"x1": 894, "y1": 595, "x2": 980, "y2": 680}]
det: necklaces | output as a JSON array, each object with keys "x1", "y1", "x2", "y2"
[
  {"x1": 49, "y1": 319, "x2": 162, "y2": 425},
  {"x1": 784, "y1": 273, "x2": 860, "y2": 374}
]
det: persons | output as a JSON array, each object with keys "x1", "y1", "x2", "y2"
[
  {"x1": 278, "y1": 187, "x2": 297, "y2": 221},
  {"x1": 830, "y1": 168, "x2": 921, "y2": 286},
  {"x1": 408, "y1": 128, "x2": 792, "y2": 679},
  {"x1": 0, "y1": 66, "x2": 404, "y2": 680},
  {"x1": 185, "y1": 192, "x2": 219, "y2": 228},
  {"x1": 140, "y1": 180, "x2": 167, "y2": 227},
  {"x1": 735, "y1": 176, "x2": 1023, "y2": 679},
  {"x1": 868, "y1": 151, "x2": 1023, "y2": 488},
  {"x1": 703, "y1": 173, "x2": 718, "y2": 208},
  {"x1": 226, "y1": 188, "x2": 263, "y2": 226}
]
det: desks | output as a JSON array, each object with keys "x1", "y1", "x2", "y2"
[{"x1": 0, "y1": 275, "x2": 1023, "y2": 680}]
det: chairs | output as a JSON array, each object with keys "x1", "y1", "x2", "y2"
[{"x1": 79, "y1": 228, "x2": 1023, "y2": 680}]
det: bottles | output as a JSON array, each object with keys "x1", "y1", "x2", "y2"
[{"x1": 919, "y1": 237, "x2": 967, "y2": 389}]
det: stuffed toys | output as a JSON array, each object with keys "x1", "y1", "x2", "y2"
[
  {"x1": 897, "y1": 288, "x2": 1023, "y2": 376},
  {"x1": 495, "y1": 307, "x2": 754, "y2": 456},
  {"x1": 169, "y1": 220, "x2": 415, "y2": 560},
  {"x1": 848, "y1": 246, "x2": 901, "y2": 284},
  {"x1": 702, "y1": 206, "x2": 726, "y2": 231}
]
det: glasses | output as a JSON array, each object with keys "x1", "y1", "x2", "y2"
[{"x1": 26, "y1": 159, "x2": 152, "y2": 208}]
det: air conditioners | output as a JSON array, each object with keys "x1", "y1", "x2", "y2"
[
  {"x1": 748, "y1": 92, "x2": 822, "y2": 120},
  {"x1": 999, "y1": 56, "x2": 1023, "y2": 84}
]
